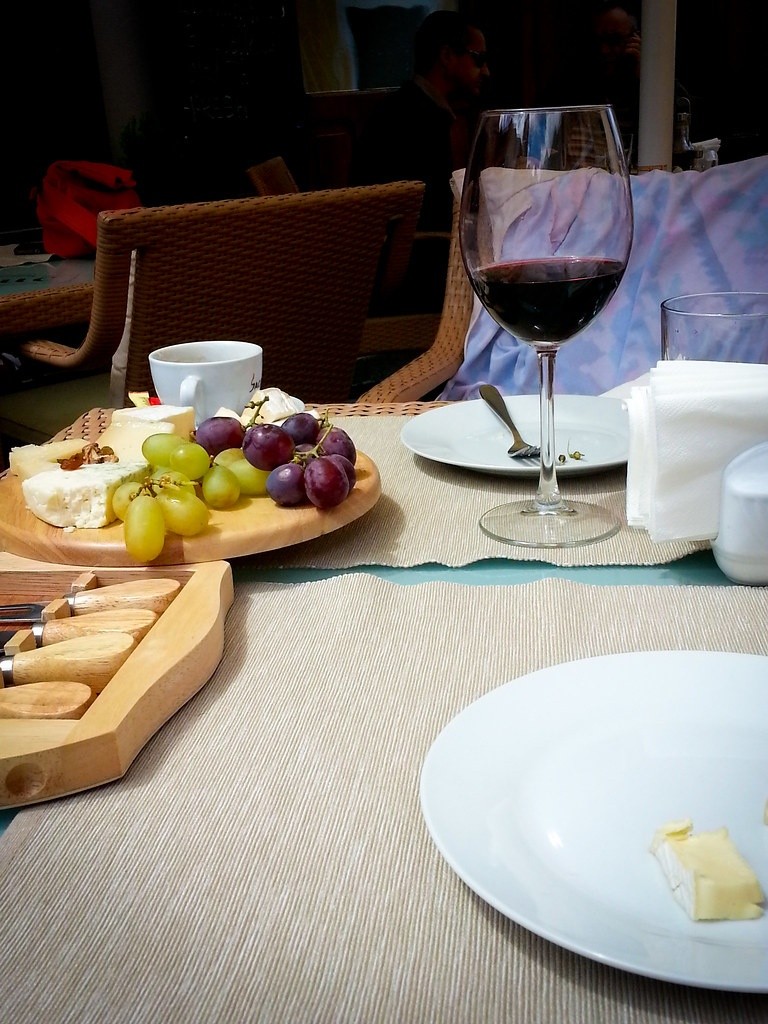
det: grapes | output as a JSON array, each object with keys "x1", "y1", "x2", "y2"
[{"x1": 113, "y1": 412, "x2": 357, "y2": 562}]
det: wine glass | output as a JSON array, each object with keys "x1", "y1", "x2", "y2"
[{"x1": 458, "y1": 104, "x2": 635, "y2": 548}]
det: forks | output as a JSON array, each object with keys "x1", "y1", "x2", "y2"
[{"x1": 478, "y1": 384, "x2": 541, "y2": 457}]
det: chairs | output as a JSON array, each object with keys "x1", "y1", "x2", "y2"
[
  {"x1": 354, "y1": 166, "x2": 493, "y2": 405},
  {"x1": 0, "y1": 176, "x2": 430, "y2": 445}
]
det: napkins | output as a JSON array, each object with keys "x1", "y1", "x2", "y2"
[{"x1": 627, "y1": 359, "x2": 767, "y2": 543}]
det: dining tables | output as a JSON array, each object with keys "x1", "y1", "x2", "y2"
[{"x1": 0, "y1": 396, "x2": 768, "y2": 1024}]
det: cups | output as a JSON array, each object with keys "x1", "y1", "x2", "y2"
[
  {"x1": 149, "y1": 341, "x2": 263, "y2": 428},
  {"x1": 660, "y1": 291, "x2": 767, "y2": 366}
]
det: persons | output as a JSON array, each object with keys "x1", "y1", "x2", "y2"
[
  {"x1": 567, "y1": 10, "x2": 691, "y2": 171},
  {"x1": 392, "y1": 8, "x2": 491, "y2": 230}
]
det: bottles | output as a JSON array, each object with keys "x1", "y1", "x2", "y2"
[{"x1": 673, "y1": 97, "x2": 695, "y2": 172}]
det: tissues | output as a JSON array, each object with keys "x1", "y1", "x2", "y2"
[{"x1": 625, "y1": 359, "x2": 768, "y2": 543}]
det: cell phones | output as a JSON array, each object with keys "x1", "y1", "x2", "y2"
[{"x1": 14, "y1": 242, "x2": 46, "y2": 255}]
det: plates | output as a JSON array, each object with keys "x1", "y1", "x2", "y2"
[
  {"x1": 419, "y1": 651, "x2": 768, "y2": 993},
  {"x1": 401, "y1": 394, "x2": 628, "y2": 475}
]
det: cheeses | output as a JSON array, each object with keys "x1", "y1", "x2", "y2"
[
  {"x1": 653, "y1": 820, "x2": 766, "y2": 921},
  {"x1": 7, "y1": 405, "x2": 195, "y2": 529},
  {"x1": 214, "y1": 387, "x2": 321, "y2": 427}
]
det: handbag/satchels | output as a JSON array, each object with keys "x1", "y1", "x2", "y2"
[{"x1": 35, "y1": 161, "x2": 144, "y2": 255}]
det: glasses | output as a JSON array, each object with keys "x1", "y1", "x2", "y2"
[{"x1": 448, "y1": 46, "x2": 487, "y2": 69}]
